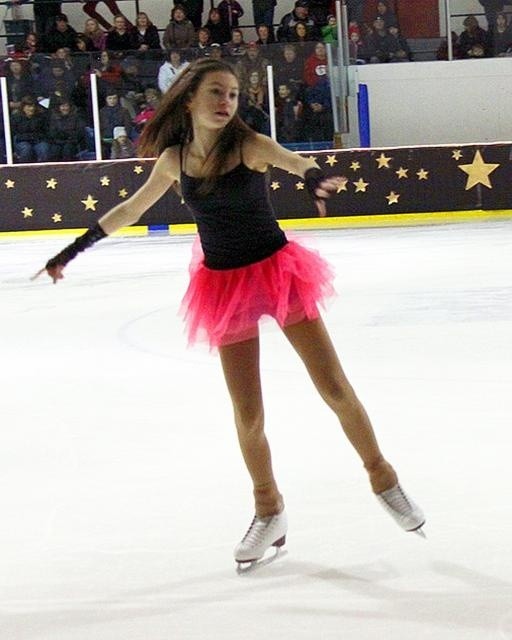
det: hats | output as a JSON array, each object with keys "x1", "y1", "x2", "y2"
[
  {"x1": 295, "y1": 0, "x2": 312, "y2": 7},
  {"x1": 113, "y1": 126, "x2": 128, "y2": 140}
]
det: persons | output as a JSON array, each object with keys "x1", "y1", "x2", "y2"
[
  {"x1": 488, "y1": 12, "x2": 512, "y2": 57},
  {"x1": 477, "y1": 1, "x2": 508, "y2": 26},
  {"x1": 28, "y1": 55, "x2": 428, "y2": 563},
  {"x1": 6, "y1": 0, "x2": 338, "y2": 163},
  {"x1": 438, "y1": 31, "x2": 460, "y2": 60},
  {"x1": 454, "y1": 14, "x2": 489, "y2": 59},
  {"x1": 346, "y1": 2, "x2": 412, "y2": 65}
]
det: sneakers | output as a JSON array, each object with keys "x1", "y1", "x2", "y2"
[
  {"x1": 235, "y1": 507, "x2": 287, "y2": 563},
  {"x1": 376, "y1": 482, "x2": 424, "y2": 531}
]
